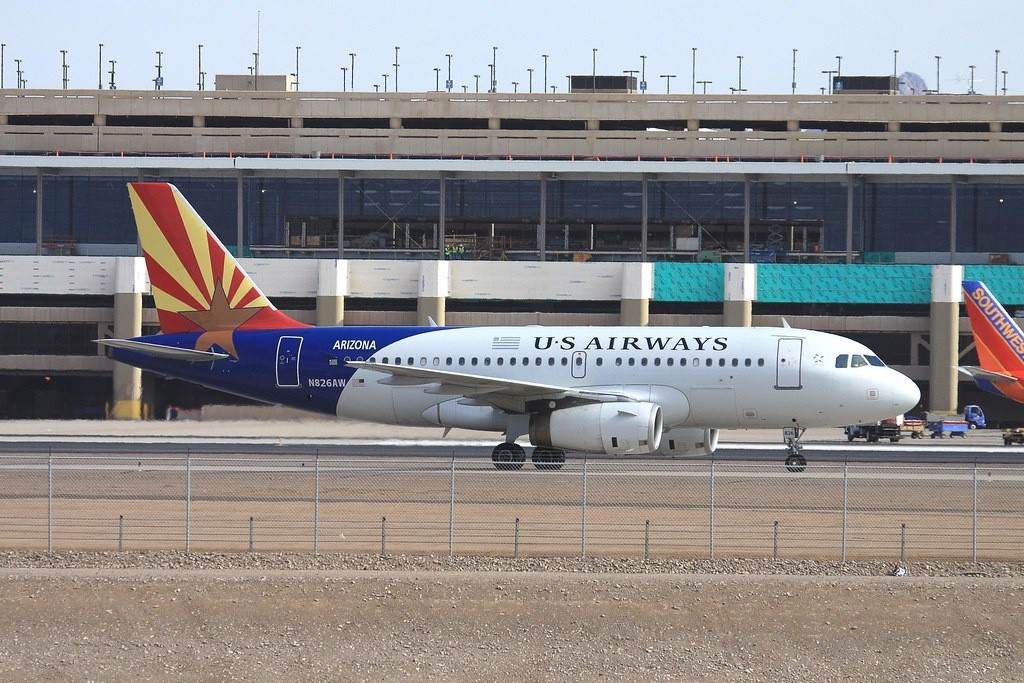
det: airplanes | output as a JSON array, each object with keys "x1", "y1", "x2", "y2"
[
  {"x1": 91, "y1": 180, "x2": 920, "y2": 472},
  {"x1": 951, "y1": 279, "x2": 1024, "y2": 406}
]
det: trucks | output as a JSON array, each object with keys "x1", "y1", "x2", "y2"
[
  {"x1": 844, "y1": 414, "x2": 904, "y2": 443},
  {"x1": 926, "y1": 405, "x2": 987, "y2": 430}
]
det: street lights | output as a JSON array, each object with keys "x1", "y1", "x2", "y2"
[
  {"x1": 692, "y1": 47, "x2": 697, "y2": 95},
  {"x1": 434, "y1": 44, "x2": 571, "y2": 102},
  {"x1": 97, "y1": 43, "x2": 105, "y2": 89},
  {"x1": 109, "y1": 59, "x2": 118, "y2": 88},
  {"x1": 591, "y1": 48, "x2": 598, "y2": 93},
  {"x1": 727, "y1": 55, "x2": 746, "y2": 103},
  {"x1": 340, "y1": 53, "x2": 355, "y2": 92},
  {"x1": 152, "y1": 51, "x2": 163, "y2": 99},
  {"x1": 635, "y1": 55, "x2": 651, "y2": 93},
  {"x1": 197, "y1": 43, "x2": 206, "y2": 90},
  {"x1": 1, "y1": 43, "x2": 7, "y2": 88},
  {"x1": 699, "y1": 81, "x2": 714, "y2": 103},
  {"x1": 290, "y1": 47, "x2": 301, "y2": 90},
  {"x1": 789, "y1": 47, "x2": 1013, "y2": 105},
  {"x1": 58, "y1": 50, "x2": 69, "y2": 98},
  {"x1": 374, "y1": 46, "x2": 400, "y2": 91},
  {"x1": 246, "y1": 51, "x2": 259, "y2": 92},
  {"x1": 13, "y1": 57, "x2": 29, "y2": 98},
  {"x1": 622, "y1": 70, "x2": 638, "y2": 94},
  {"x1": 659, "y1": 74, "x2": 676, "y2": 95}
]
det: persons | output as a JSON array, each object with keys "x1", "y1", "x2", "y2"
[
  {"x1": 916, "y1": 404, "x2": 929, "y2": 419},
  {"x1": 444, "y1": 244, "x2": 465, "y2": 260}
]
